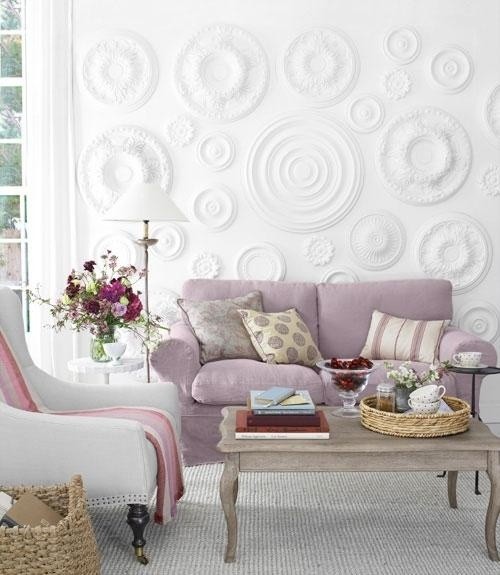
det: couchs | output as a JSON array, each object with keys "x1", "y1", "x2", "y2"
[{"x1": 152, "y1": 277, "x2": 498, "y2": 468}]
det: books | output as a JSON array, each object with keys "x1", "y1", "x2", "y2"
[
  {"x1": 1, "y1": 491, "x2": 64, "y2": 530},
  {"x1": 236, "y1": 387, "x2": 332, "y2": 441}
]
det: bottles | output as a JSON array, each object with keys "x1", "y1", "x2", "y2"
[{"x1": 378, "y1": 386, "x2": 394, "y2": 411}]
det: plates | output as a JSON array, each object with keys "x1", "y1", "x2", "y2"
[{"x1": 450, "y1": 363, "x2": 490, "y2": 370}]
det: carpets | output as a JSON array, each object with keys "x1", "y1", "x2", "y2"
[{"x1": 80, "y1": 464, "x2": 500, "y2": 573}]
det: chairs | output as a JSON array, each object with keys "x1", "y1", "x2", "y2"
[{"x1": 2, "y1": 287, "x2": 185, "y2": 567}]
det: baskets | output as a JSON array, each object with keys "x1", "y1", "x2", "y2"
[
  {"x1": 0, "y1": 473, "x2": 103, "y2": 575},
  {"x1": 359, "y1": 393, "x2": 472, "y2": 438}
]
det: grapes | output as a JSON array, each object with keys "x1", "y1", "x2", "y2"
[{"x1": 325, "y1": 356, "x2": 373, "y2": 392}]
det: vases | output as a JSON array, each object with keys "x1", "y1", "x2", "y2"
[{"x1": 88, "y1": 323, "x2": 124, "y2": 364}]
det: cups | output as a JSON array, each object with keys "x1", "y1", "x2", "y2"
[
  {"x1": 409, "y1": 384, "x2": 445, "y2": 414},
  {"x1": 454, "y1": 352, "x2": 481, "y2": 364}
]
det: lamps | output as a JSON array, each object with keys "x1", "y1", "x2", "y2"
[{"x1": 107, "y1": 185, "x2": 188, "y2": 340}]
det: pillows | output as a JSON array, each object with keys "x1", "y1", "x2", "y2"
[
  {"x1": 358, "y1": 305, "x2": 451, "y2": 366},
  {"x1": 176, "y1": 291, "x2": 325, "y2": 366}
]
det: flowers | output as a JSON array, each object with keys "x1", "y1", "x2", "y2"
[
  {"x1": 384, "y1": 357, "x2": 450, "y2": 388},
  {"x1": 28, "y1": 252, "x2": 170, "y2": 361}
]
